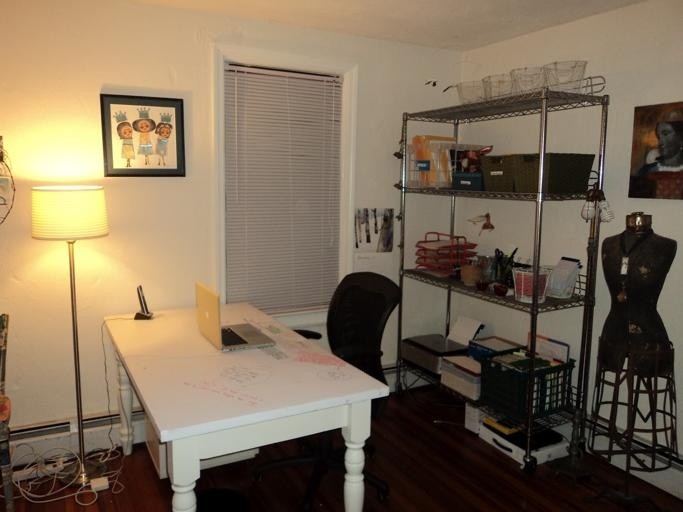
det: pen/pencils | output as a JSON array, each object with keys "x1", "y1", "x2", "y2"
[
  {"x1": 510, "y1": 352, "x2": 530, "y2": 356},
  {"x1": 505, "y1": 248, "x2": 518, "y2": 268}
]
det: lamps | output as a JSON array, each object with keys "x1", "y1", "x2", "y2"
[{"x1": 31, "y1": 184, "x2": 112, "y2": 487}]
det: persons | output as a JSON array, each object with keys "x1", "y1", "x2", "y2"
[
  {"x1": 631, "y1": 107, "x2": 683, "y2": 199},
  {"x1": 601, "y1": 211, "x2": 678, "y2": 373}
]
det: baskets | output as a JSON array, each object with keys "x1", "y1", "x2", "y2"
[
  {"x1": 407, "y1": 144, "x2": 457, "y2": 191},
  {"x1": 481, "y1": 357, "x2": 577, "y2": 419}
]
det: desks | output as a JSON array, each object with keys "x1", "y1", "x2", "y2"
[{"x1": 104, "y1": 301, "x2": 390, "y2": 512}]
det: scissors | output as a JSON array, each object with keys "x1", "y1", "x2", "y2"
[{"x1": 495, "y1": 248, "x2": 504, "y2": 262}]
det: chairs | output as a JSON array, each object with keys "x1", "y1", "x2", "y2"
[{"x1": 293, "y1": 272, "x2": 400, "y2": 501}]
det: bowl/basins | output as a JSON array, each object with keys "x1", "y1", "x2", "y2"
[
  {"x1": 455, "y1": 80, "x2": 484, "y2": 103},
  {"x1": 511, "y1": 66, "x2": 548, "y2": 94},
  {"x1": 545, "y1": 60, "x2": 586, "y2": 93},
  {"x1": 481, "y1": 76, "x2": 513, "y2": 100}
]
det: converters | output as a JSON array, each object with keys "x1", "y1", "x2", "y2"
[{"x1": 90, "y1": 476, "x2": 109, "y2": 492}]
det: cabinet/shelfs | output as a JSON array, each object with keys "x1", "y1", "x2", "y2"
[{"x1": 400, "y1": 90, "x2": 608, "y2": 465}]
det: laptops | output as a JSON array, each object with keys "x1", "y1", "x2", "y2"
[{"x1": 195, "y1": 281, "x2": 276, "y2": 352}]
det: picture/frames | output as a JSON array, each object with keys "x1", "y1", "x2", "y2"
[{"x1": 99, "y1": 93, "x2": 186, "y2": 179}]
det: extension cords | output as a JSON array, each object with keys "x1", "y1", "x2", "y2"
[{"x1": 12, "y1": 461, "x2": 64, "y2": 482}]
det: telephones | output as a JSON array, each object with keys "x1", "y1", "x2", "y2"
[{"x1": 134, "y1": 285, "x2": 153, "y2": 320}]
